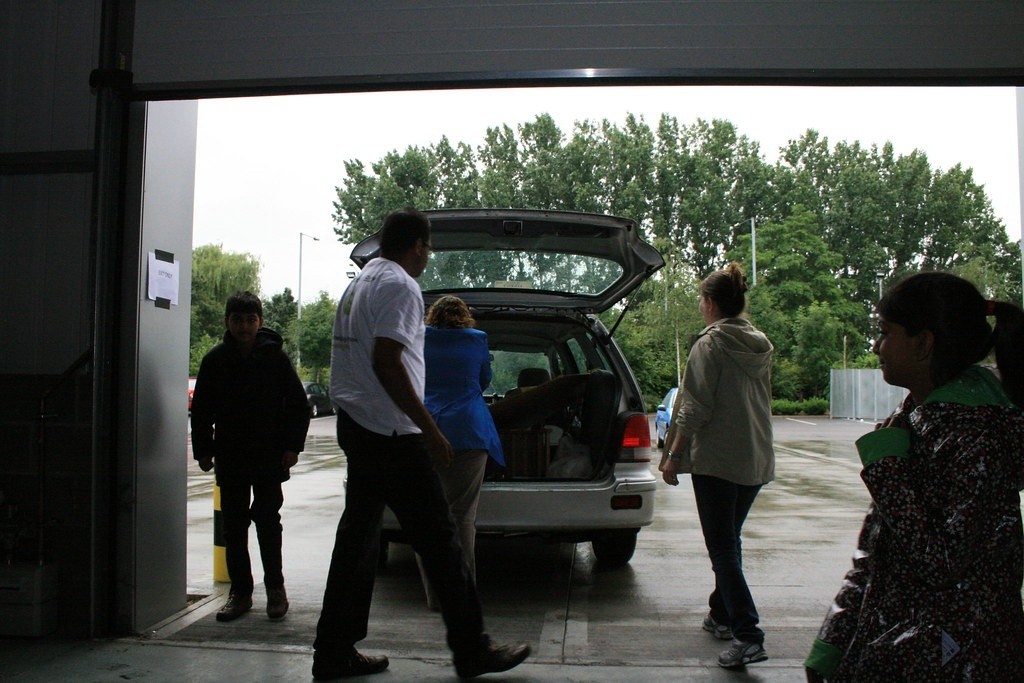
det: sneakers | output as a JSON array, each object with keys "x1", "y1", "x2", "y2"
[
  {"x1": 215, "y1": 591, "x2": 252, "y2": 621},
  {"x1": 703, "y1": 614, "x2": 735, "y2": 639},
  {"x1": 266, "y1": 582, "x2": 289, "y2": 621},
  {"x1": 718, "y1": 636, "x2": 768, "y2": 668}
]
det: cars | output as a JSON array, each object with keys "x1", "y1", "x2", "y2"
[
  {"x1": 188, "y1": 376, "x2": 198, "y2": 412},
  {"x1": 302, "y1": 381, "x2": 336, "y2": 417},
  {"x1": 655, "y1": 387, "x2": 679, "y2": 448}
]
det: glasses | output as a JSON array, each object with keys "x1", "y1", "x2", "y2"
[{"x1": 411, "y1": 239, "x2": 434, "y2": 255}]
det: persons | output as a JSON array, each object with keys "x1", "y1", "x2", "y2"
[
  {"x1": 802, "y1": 271, "x2": 1024, "y2": 682},
  {"x1": 416, "y1": 294, "x2": 506, "y2": 613},
  {"x1": 311, "y1": 208, "x2": 529, "y2": 680},
  {"x1": 660, "y1": 259, "x2": 778, "y2": 671},
  {"x1": 191, "y1": 292, "x2": 312, "y2": 620}
]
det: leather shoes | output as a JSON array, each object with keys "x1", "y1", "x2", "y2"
[
  {"x1": 312, "y1": 647, "x2": 389, "y2": 680},
  {"x1": 454, "y1": 643, "x2": 529, "y2": 679}
]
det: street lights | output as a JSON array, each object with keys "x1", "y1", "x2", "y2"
[
  {"x1": 732, "y1": 216, "x2": 758, "y2": 287},
  {"x1": 296, "y1": 232, "x2": 320, "y2": 373}
]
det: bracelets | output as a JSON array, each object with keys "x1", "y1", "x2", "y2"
[{"x1": 665, "y1": 449, "x2": 683, "y2": 463}]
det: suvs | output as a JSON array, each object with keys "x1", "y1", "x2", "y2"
[{"x1": 350, "y1": 207, "x2": 658, "y2": 570}]
